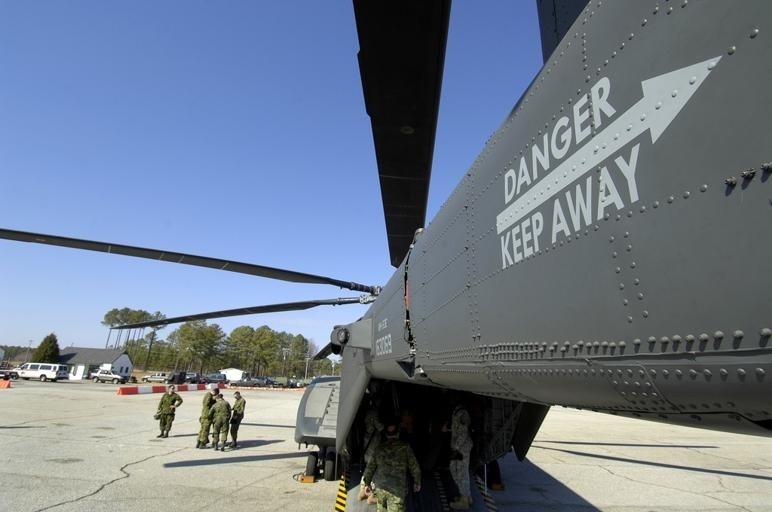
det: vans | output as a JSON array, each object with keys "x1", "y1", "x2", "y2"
[{"x1": 13, "y1": 362, "x2": 69, "y2": 381}]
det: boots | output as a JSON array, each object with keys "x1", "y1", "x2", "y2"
[
  {"x1": 448, "y1": 494, "x2": 474, "y2": 510},
  {"x1": 356, "y1": 483, "x2": 378, "y2": 505},
  {"x1": 195, "y1": 439, "x2": 239, "y2": 451},
  {"x1": 156, "y1": 430, "x2": 169, "y2": 438}
]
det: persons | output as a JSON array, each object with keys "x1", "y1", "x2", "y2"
[
  {"x1": 156, "y1": 384, "x2": 183, "y2": 438},
  {"x1": 196, "y1": 388, "x2": 247, "y2": 451},
  {"x1": 355, "y1": 392, "x2": 475, "y2": 512}
]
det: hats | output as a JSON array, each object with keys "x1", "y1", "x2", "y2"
[{"x1": 383, "y1": 424, "x2": 401, "y2": 434}]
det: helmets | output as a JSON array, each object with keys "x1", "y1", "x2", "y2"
[{"x1": 154, "y1": 413, "x2": 162, "y2": 419}]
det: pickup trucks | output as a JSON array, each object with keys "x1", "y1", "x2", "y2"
[
  {"x1": 142, "y1": 372, "x2": 303, "y2": 388},
  {"x1": 90, "y1": 368, "x2": 128, "y2": 384}
]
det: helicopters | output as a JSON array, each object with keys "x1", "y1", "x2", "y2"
[{"x1": 0, "y1": 2, "x2": 772, "y2": 512}]
